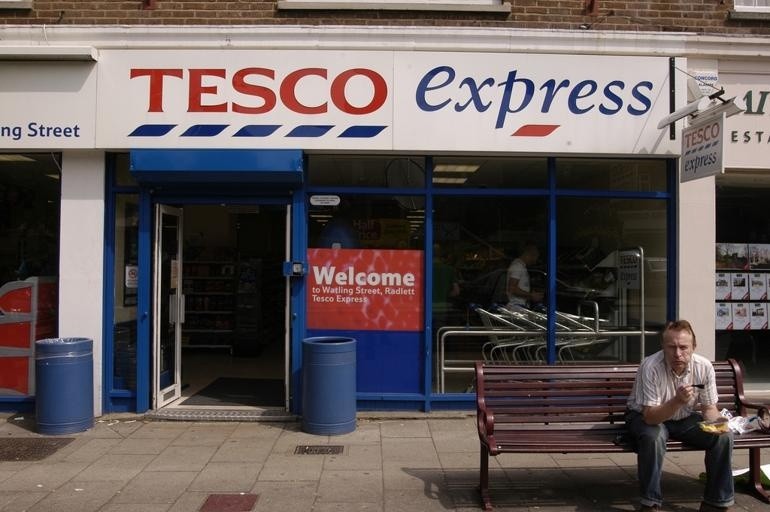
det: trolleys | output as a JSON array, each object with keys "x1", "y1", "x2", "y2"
[{"x1": 464, "y1": 303, "x2": 609, "y2": 393}]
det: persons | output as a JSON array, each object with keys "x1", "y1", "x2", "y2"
[
  {"x1": 622, "y1": 320, "x2": 737, "y2": 512},
  {"x1": 432, "y1": 242, "x2": 461, "y2": 380},
  {"x1": 504, "y1": 243, "x2": 545, "y2": 361}
]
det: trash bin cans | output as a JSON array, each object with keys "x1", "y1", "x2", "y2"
[
  {"x1": 301, "y1": 336, "x2": 357, "y2": 436},
  {"x1": 35, "y1": 338, "x2": 93, "y2": 434}
]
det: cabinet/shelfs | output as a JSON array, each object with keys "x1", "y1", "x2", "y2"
[{"x1": 182, "y1": 259, "x2": 259, "y2": 357}]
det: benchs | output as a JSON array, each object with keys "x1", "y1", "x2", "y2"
[{"x1": 473, "y1": 357, "x2": 769, "y2": 511}]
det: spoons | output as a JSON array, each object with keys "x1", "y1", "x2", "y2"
[{"x1": 692, "y1": 384, "x2": 704, "y2": 389}]
